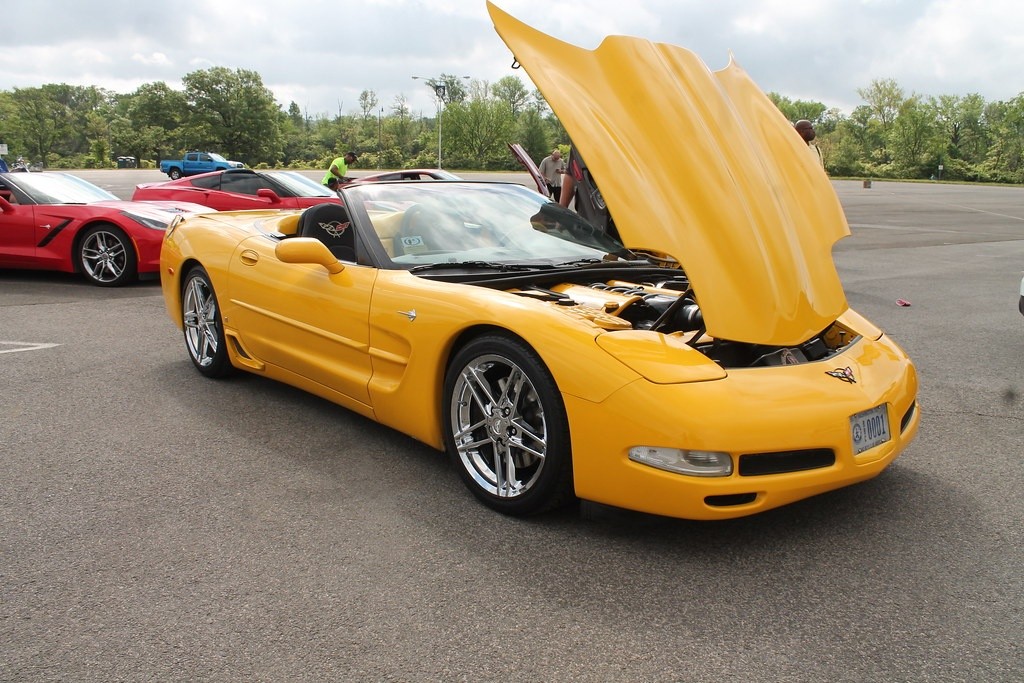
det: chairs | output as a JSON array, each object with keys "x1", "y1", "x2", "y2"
[
  {"x1": 295, "y1": 203, "x2": 358, "y2": 261},
  {"x1": 394, "y1": 202, "x2": 457, "y2": 257}
]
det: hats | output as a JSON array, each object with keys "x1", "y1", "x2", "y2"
[{"x1": 348, "y1": 152, "x2": 359, "y2": 163}]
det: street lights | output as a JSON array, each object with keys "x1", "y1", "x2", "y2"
[{"x1": 412, "y1": 76, "x2": 471, "y2": 169}]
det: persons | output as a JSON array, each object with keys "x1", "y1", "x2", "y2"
[
  {"x1": 321, "y1": 151, "x2": 360, "y2": 191},
  {"x1": 795, "y1": 119, "x2": 825, "y2": 172},
  {"x1": 327, "y1": 176, "x2": 339, "y2": 190},
  {"x1": 538, "y1": 147, "x2": 565, "y2": 204},
  {"x1": 559, "y1": 146, "x2": 607, "y2": 232}
]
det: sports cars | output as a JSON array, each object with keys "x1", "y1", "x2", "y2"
[
  {"x1": 131, "y1": 166, "x2": 347, "y2": 213},
  {"x1": 156, "y1": 0, "x2": 922, "y2": 523},
  {"x1": 0, "y1": 170, "x2": 220, "y2": 287},
  {"x1": 328, "y1": 140, "x2": 551, "y2": 199}
]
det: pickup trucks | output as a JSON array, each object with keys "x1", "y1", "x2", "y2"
[{"x1": 159, "y1": 151, "x2": 246, "y2": 180}]
